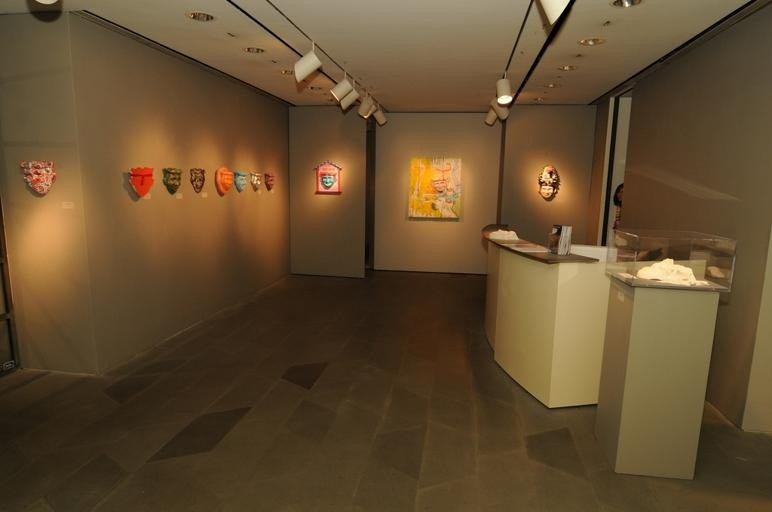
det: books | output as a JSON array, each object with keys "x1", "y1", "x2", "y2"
[{"x1": 547, "y1": 224, "x2": 574, "y2": 257}]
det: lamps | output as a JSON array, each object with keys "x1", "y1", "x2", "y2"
[{"x1": 293, "y1": 0, "x2": 572, "y2": 126}]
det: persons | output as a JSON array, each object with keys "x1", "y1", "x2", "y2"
[
  {"x1": 190, "y1": 168, "x2": 205, "y2": 194},
  {"x1": 163, "y1": 168, "x2": 183, "y2": 193},
  {"x1": 538, "y1": 165, "x2": 560, "y2": 198},
  {"x1": 612, "y1": 182, "x2": 623, "y2": 234},
  {"x1": 320, "y1": 166, "x2": 337, "y2": 187}
]
